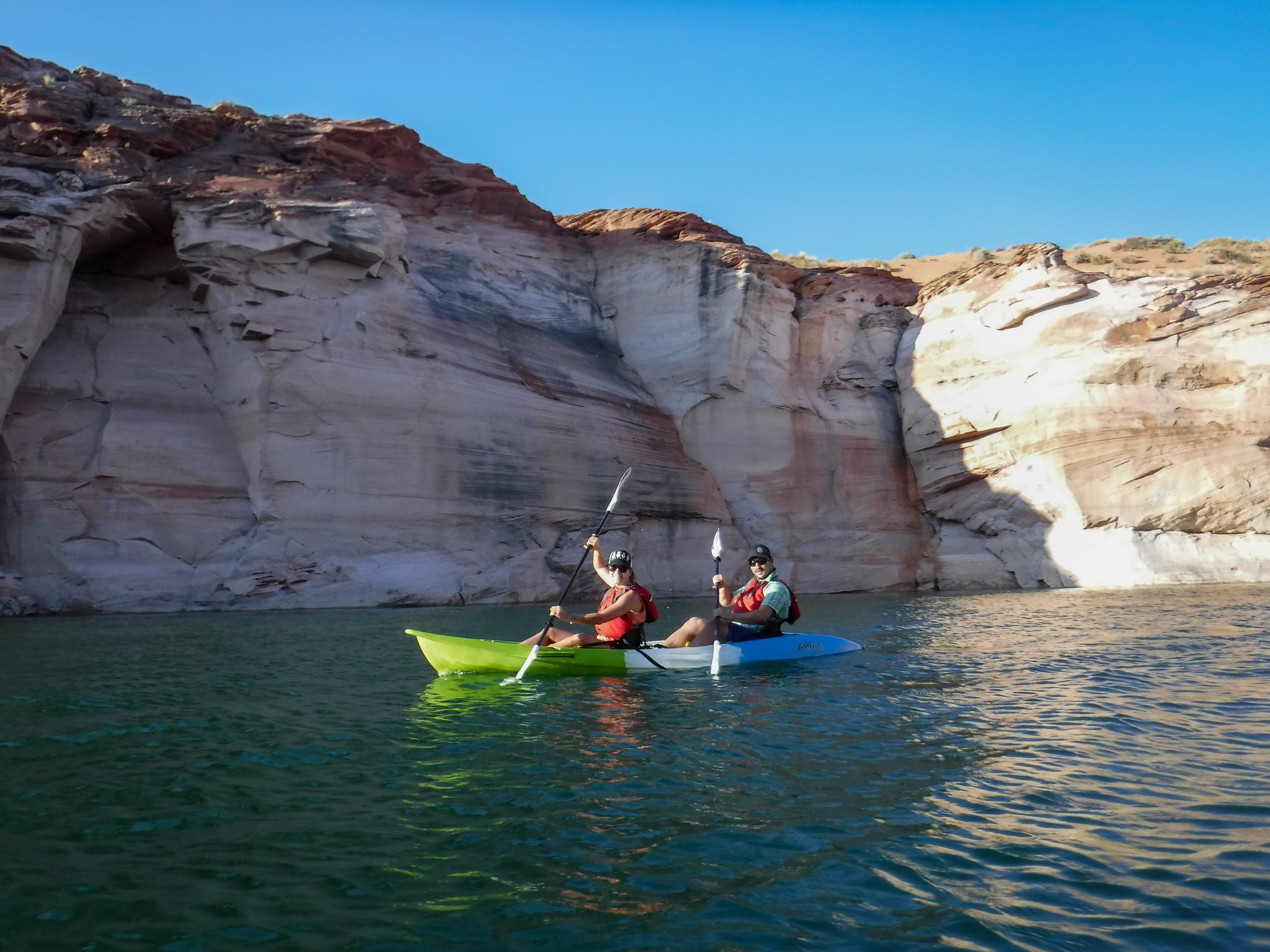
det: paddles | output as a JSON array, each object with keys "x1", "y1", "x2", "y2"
[
  {"x1": 515, "y1": 466, "x2": 632, "y2": 680},
  {"x1": 711, "y1": 527, "x2": 721, "y2": 677}
]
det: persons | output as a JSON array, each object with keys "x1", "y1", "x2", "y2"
[
  {"x1": 516, "y1": 536, "x2": 646, "y2": 649},
  {"x1": 641, "y1": 543, "x2": 791, "y2": 649}
]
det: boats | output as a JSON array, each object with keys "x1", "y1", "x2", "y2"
[{"x1": 404, "y1": 628, "x2": 861, "y2": 675}]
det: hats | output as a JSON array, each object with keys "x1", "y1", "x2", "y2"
[
  {"x1": 608, "y1": 550, "x2": 631, "y2": 570},
  {"x1": 747, "y1": 544, "x2": 772, "y2": 560}
]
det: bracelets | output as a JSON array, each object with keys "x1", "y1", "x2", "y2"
[{"x1": 570, "y1": 613, "x2": 575, "y2": 624}]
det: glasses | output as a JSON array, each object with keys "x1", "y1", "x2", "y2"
[
  {"x1": 608, "y1": 564, "x2": 630, "y2": 573},
  {"x1": 748, "y1": 558, "x2": 771, "y2": 566}
]
